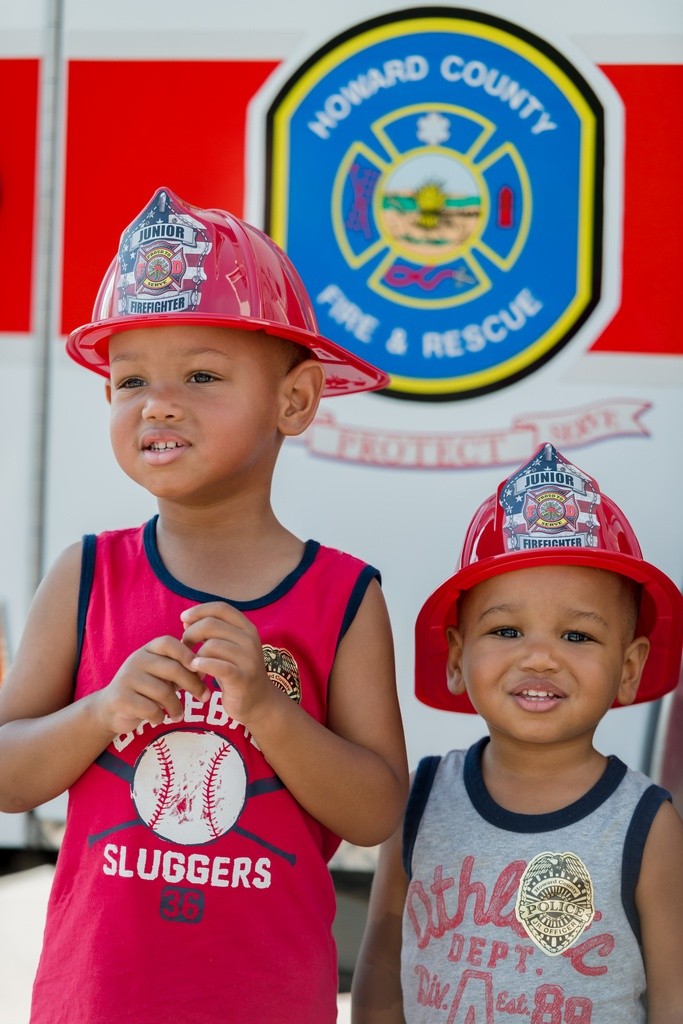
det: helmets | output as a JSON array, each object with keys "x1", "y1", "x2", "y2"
[
  {"x1": 64, "y1": 208, "x2": 390, "y2": 399},
  {"x1": 410, "y1": 440, "x2": 683, "y2": 716}
]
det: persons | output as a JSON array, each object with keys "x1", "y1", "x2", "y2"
[
  {"x1": 0, "y1": 185, "x2": 410, "y2": 1024},
  {"x1": 350, "y1": 442, "x2": 683, "y2": 1024}
]
